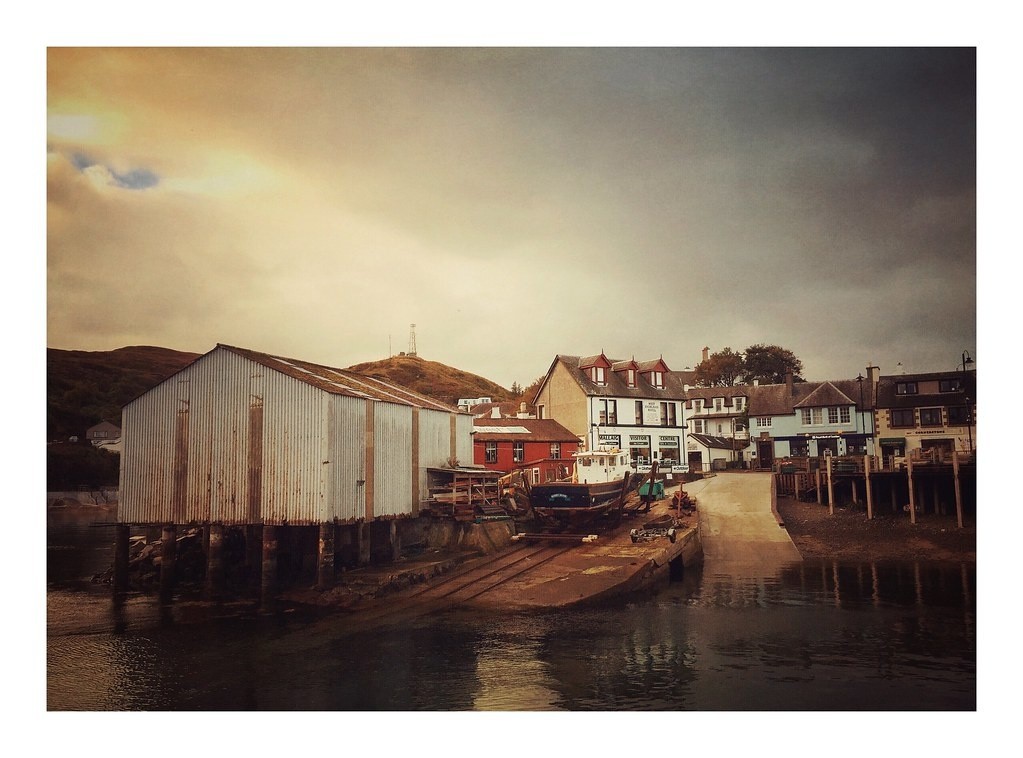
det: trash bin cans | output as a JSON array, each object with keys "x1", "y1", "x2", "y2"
[{"x1": 640, "y1": 479, "x2": 665, "y2": 499}]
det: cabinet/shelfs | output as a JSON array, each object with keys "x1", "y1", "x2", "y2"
[{"x1": 428, "y1": 485, "x2": 498, "y2": 503}]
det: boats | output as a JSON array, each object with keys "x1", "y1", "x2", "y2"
[{"x1": 530, "y1": 446, "x2": 638, "y2": 524}]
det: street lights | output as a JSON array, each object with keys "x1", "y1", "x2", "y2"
[
  {"x1": 855, "y1": 372, "x2": 868, "y2": 454},
  {"x1": 962, "y1": 349, "x2": 974, "y2": 452}
]
